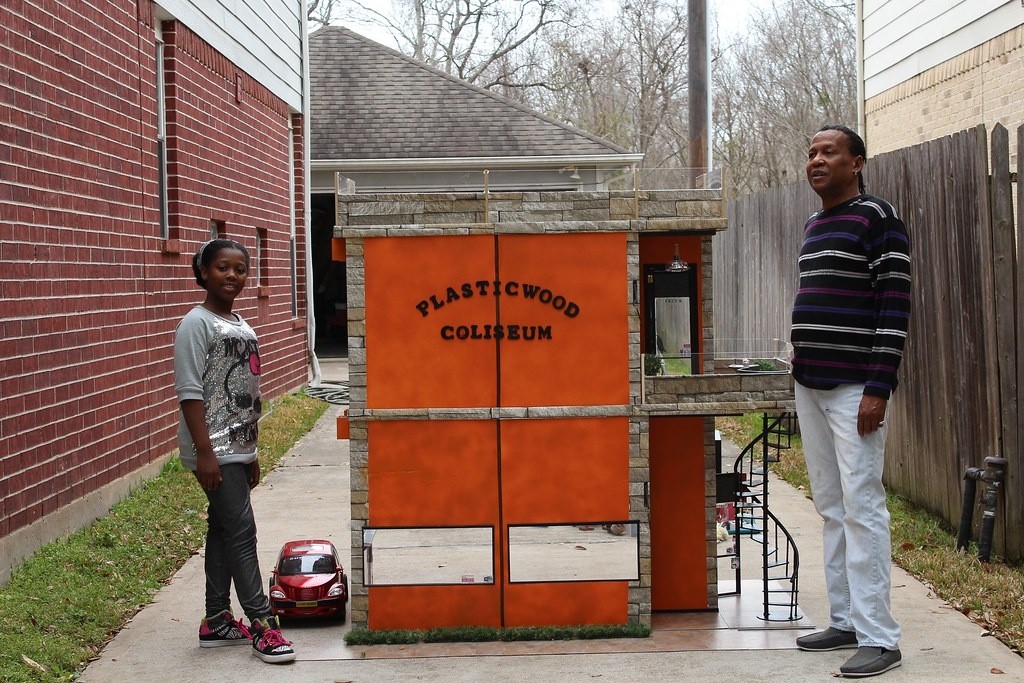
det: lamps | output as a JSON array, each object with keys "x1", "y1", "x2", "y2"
[{"x1": 666, "y1": 242, "x2": 692, "y2": 272}]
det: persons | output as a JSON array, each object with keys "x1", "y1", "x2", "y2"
[
  {"x1": 174, "y1": 238, "x2": 296, "y2": 663},
  {"x1": 790, "y1": 125, "x2": 912, "y2": 677}
]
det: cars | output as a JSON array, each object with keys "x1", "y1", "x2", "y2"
[{"x1": 269, "y1": 540, "x2": 348, "y2": 626}]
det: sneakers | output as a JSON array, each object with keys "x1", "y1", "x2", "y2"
[
  {"x1": 198, "y1": 606, "x2": 254, "y2": 647},
  {"x1": 251, "y1": 615, "x2": 296, "y2": 662},
  {"x1": 796, "y1": 627, "x2": 858, "y2": 651},
  {"x1": 839, "y1": 646, "x2": 902, "y2": 675}
]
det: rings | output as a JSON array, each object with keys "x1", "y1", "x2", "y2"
[{"x1": 879, "y1": 421, "x2": 884, "y2": 427}]
